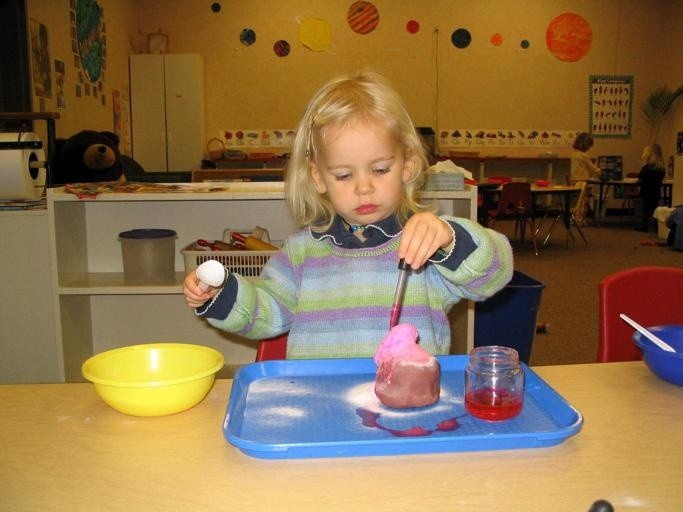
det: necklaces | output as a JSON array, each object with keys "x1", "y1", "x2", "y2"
[{"x1": 341, "y1": 216, "x2": 369, "y2": 234}]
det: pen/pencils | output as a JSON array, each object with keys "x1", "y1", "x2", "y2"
[{"x1": 390, "y1": 257, "x2": 410, "y2": 330}]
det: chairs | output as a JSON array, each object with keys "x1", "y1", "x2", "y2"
[
  {"x1": 621, "y1": 173, "x2": 640, "y2": 209},
  {"x1": 479, "y1": 177, "x2": 588, "y2": 257}
]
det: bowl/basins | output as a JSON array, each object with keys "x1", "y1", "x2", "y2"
[
  {"x1": 80, "y1": 341, "x2": 225, "y2": 418},
  {"x1": 632, "y1": 325, "x2": 682, "y2": 386}
]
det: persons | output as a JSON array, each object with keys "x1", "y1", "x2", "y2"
[
  {"x1": 179, "y1": 69, "x2": 514, "y2": 360},
  {"x1": 567, "y1": 132, "x2": 602, "y2": 226},
  {"x1": 638, "y1": 141, "x2": 666, "y2": 231}
]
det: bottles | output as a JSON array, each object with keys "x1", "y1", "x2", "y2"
[{"x1": 463, "y1": 345, "x2": 527, "y2": 423}]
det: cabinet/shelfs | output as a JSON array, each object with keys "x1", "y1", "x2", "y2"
[
  {"x1": 129, "y1": 54, "x2": 205, "y2": 174},
  {"x1": 433, "y1": 157, "x2": 572, "y2": 234}
]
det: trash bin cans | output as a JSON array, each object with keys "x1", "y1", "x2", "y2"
[
  {"x1": 474, "y1": 270, "x2": 547, "y2": 367},
  {"x1": 655, "y1": 207, "x2": 676, "y2": 239}
]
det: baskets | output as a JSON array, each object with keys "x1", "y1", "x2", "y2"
[{"x1": 180, "y1": 229, "x2": 283, "y2": 283}]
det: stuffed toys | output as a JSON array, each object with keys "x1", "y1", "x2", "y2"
[{"x1": 45, "y1": 130, "x2": 145, "y2": 184}]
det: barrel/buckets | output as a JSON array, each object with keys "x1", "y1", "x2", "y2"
[{"x1": 117, "y1": 229, "x2": 178, "y2": 280}]
